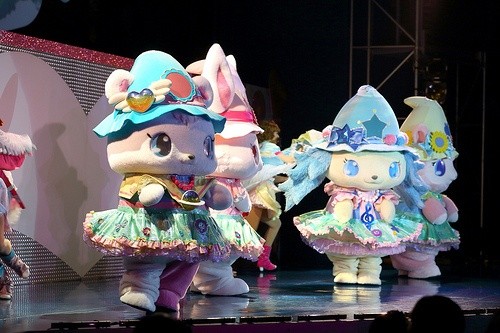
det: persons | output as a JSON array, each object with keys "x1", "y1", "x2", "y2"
[
  {"x1": 367, "y1": 295, "x2": 466, "y2": 333},
  {"x1": 231, "y1": 119, "x2": 291, "y2": 276}
]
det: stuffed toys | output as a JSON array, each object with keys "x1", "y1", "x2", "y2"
[
  {"x1": 0, "y1": 119, "x2": 37, "y2": 300},
  {"x1": 389, "y1": 97, "x2": 460, "y2": 279},
  {"x1": 187, "y1": 43, "x2": 266, "y2": 297},
  {"x1": 82, "y1": 50, "x2": 232, "y2": 312},
  {"x1": 279, "y1": 85, "x2": 424, "y2": 286}
]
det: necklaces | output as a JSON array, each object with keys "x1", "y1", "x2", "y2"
[
  {"x1": 354, "y1": 188, "x2": 380, "y2": 202},
  {"x1": 170, "y1": 174, "x2": 195, "y2": 191}
]
